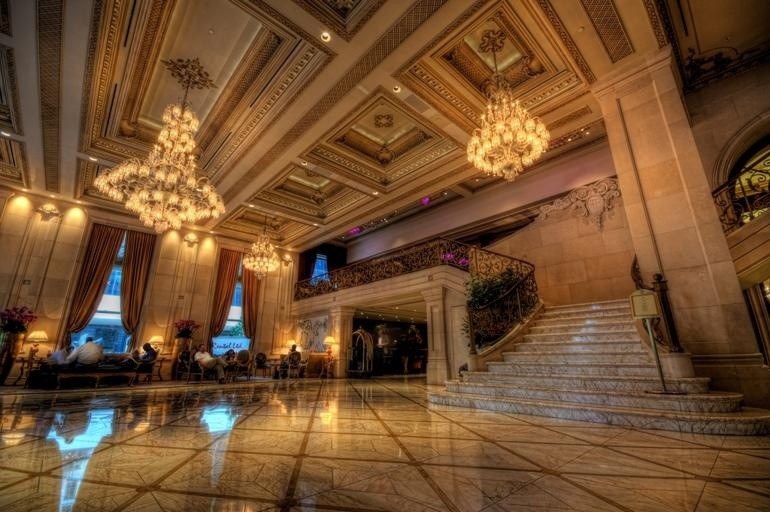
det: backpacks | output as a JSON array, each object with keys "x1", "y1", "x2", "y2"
[{"x1": 272, "y1": 367, "x2": 280, "y2": 380}]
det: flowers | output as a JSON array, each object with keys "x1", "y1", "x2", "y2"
[
  {"x1": 441, "y1": 252, "x2": 470, "y2": 266},
  {"x1": 0, "y1": 305, "x2": 39, "y2": 333},
  {"x1": 173, "y1": 319, "x2": 202, "y2": 338}
]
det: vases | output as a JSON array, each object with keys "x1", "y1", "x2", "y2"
[
  {"x1": 0, "y1": 331, "x2": 26, "y2": 386},
  {"x1": 445, "y1": 262, "x2": 468, "y2": 272},
  {"x1": 170, "y1": 338, "x2": 193, "y2": 381}
]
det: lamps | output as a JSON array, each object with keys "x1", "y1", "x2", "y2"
[
  {"x1": 465, "y1": 35, "x2": 555, "y2": 188},
  {"x1": 24, "y1": 329, "x2": 49, "y2": 355},
  {"x1": 148, "y1": 335, "x2": 166, "y2": 356},
  {"x1": 92, "y1": 66, "x2": 229, "y2": 233},
  {"x1": 323, "y1": 336, "x2": 337, "y2": 355},
  {"x1": 286, "y1": 339, "x2": 295, "y2": 352},
  {"x1": 240, "y1": 207, "x2": 282, "y2": 282}
]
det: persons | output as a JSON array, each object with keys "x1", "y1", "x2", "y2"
[
  {"x1": 67, "y1": 337, "x2": 104, "y2": 374},
  {"x1": 195, "y1": 344, "x2": 227, "y2": 384},
  {"x1": 223, "y1": 349, "x2": 235, "y2": 361},
  {"x1": 128, "y1": 342, "x2": 155, "y2": 369},
  {"x1": 46, "y1": 347, "x2": 74, "y2": 375},
  {"x1": 284, "y1": 344, "x2": 297, "y2": 361}
]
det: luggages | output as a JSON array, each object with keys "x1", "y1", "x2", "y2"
[{"x1": 280, "y1": 361, "x2": 289, "y2": 379}]
